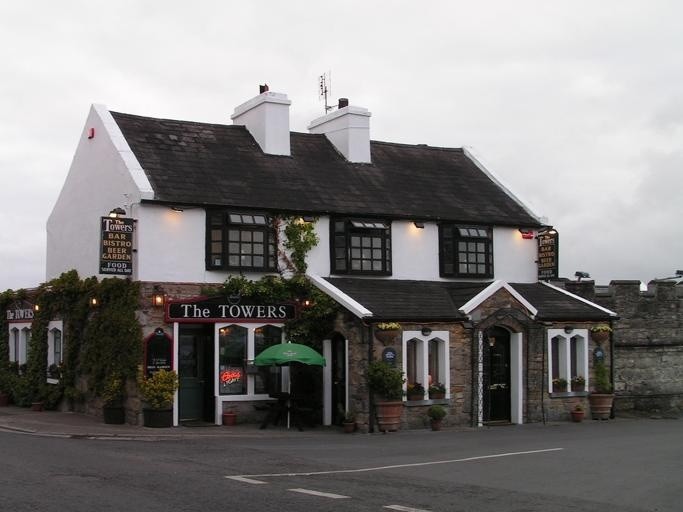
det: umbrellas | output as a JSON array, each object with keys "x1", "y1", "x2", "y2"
[{"x1": 255, "y1": 341, "x2": 325, "y2": 431}]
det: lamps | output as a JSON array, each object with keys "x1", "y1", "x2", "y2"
[{"x1": 149, "y1": 283, "x2": 167, "y2": 307}]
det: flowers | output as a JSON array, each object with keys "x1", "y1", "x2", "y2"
[
  {"x1": 588, "y1": 325, "x2": 613, "y2": 334},
  {"x1": 377, "y1": 321, "x2": 401, "y2": 332}
]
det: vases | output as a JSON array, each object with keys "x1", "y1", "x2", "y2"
[
  {"x1": 221, "y1": 412, "x2": 236, "y2": 426},
  {"x1": 343, "y1": 414, "x2": 356, "y2": 432}
]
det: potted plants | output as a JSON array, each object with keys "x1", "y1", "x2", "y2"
[
  {"x1": 366, "y1": 361, "x2": 445, "y2": 431},
  {"x1": 99, "y1": 369, "x2": 178, "y2": 427},
  {"x1": 551, "y1": 356, "x2": 615, "y2": 423}
]
